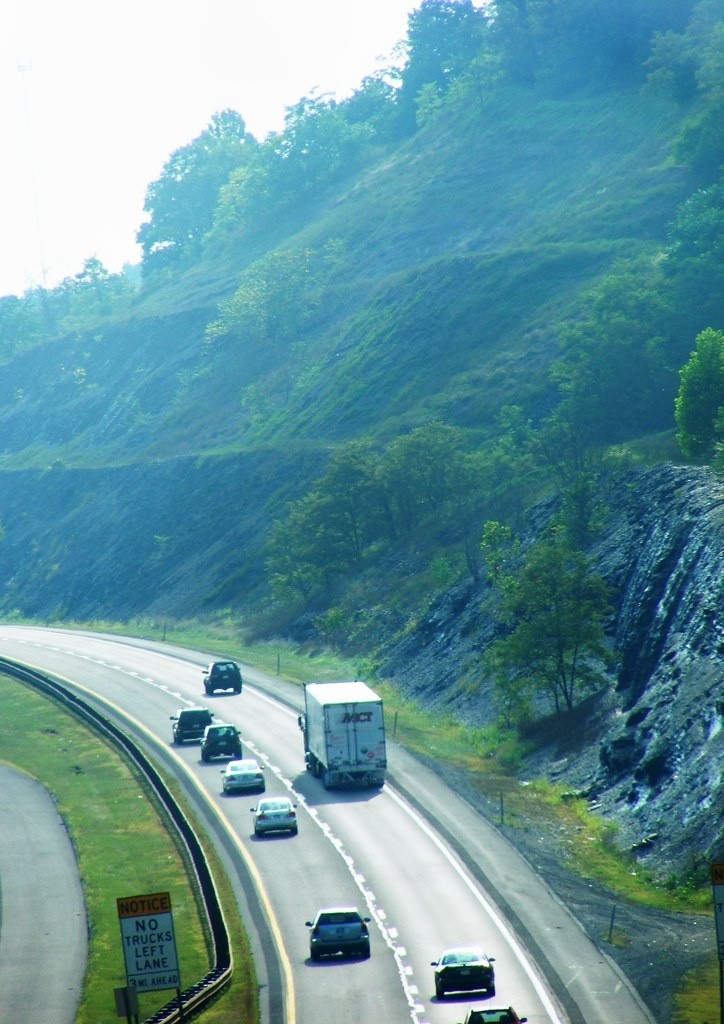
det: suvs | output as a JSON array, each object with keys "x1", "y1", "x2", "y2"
[
  {"x1": 169, "y1": 706, "x2": 215, "y2": 745},
  {"x1": 201, "y1": 724, "x2": 243, "y2": 762},
  {"x1": 202, "y1": 659, "x2": 244, "y2": 696}
]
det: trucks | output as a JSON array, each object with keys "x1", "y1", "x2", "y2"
[{"x1": 297, "y1": 681, "x2": 386, "y2": 791}]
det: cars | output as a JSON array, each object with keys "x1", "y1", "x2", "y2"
[
  {"x1": 457, "y1": 1007, "x2": 527, "y2": 1024},
  {"x1": 306, "y1": 906, "x2": 371, "y2": 962},
  {"x1": 250, "y1": 796, "x2": 298, "y2": 836},
  {"x1": 430, "y1": 949, "x2": 496, "y2": 996},
  {"x1": 220, "y1": 759, "x2": 266, "y2": 794}
]
